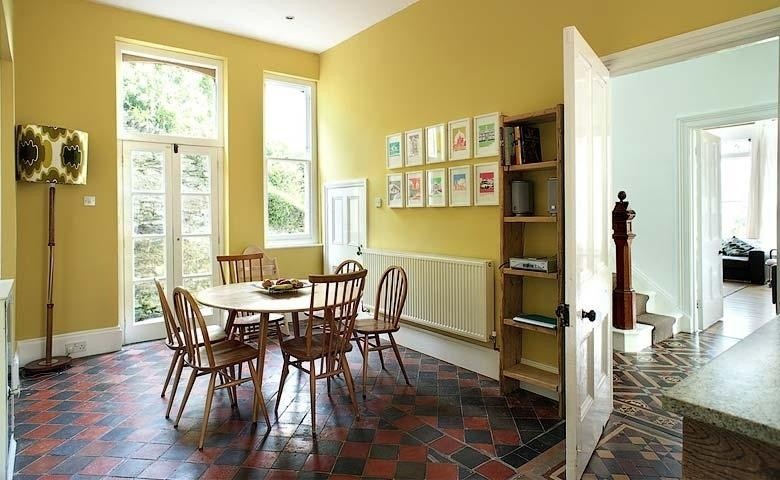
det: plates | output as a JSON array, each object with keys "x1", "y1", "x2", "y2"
[{"x1": 250, "y1": 279, "x2": 318, "y2": 292}]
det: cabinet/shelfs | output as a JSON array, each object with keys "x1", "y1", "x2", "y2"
[{"x1": 498, "y1": 104, "x2": 565, "y2": 418}]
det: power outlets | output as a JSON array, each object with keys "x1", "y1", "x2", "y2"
[
  {"x1": 66, "y1": 342, "x2": 87, "y2": 353},
  {"x1": 83, "y1": 196, "x2": 95, "y2": 205}
]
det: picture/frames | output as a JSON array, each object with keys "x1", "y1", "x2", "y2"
[{"x1": 385, "y1": 111, "x2": 502, "y2": 209}]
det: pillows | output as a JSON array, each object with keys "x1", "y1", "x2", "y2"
[{"x1": 721, "y1": 236, "x2": 758, "y2": 256}]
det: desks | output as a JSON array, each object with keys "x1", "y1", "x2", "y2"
[{"x1": 195, "y1": 280, "x2": 365, "y2": 450}]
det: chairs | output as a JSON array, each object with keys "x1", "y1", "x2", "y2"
[
  {"x1": 155, "y1": 278, "x2": 271, "y2": 450},
  {"x1": 275, "y1": 269, "x2": 367, "y2": 434},
  {"x1": 217, "y1": 253, "x2": 285, "y2": 386},
  {"x1": 305, "y1": 261, "x2": 409, "y2": 395}
]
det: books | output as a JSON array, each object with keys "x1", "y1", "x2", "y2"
[{"x1": 503, "y1": 123, "x2": 542, "y2": 165}]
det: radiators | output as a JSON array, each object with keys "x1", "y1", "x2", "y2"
[{"x1": 361, "y1": 248, "x2": 494, "y2": 343}]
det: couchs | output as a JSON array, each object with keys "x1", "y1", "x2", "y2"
[
  {"x1": 766, "y1": 249, "x2": 777, "y2": 304},
  {"x1": 722, "y1": 247, "x2": 771, "y2": 284}
]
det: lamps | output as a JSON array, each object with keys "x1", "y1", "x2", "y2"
[{"x1": 12, "y1": 123, "x2": 89, "y2": 370}]
